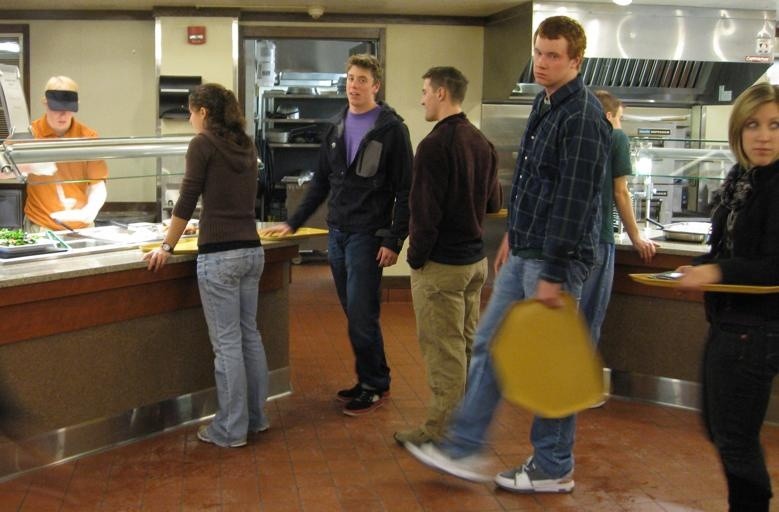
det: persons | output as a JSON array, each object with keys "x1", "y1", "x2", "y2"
[
  {"x1": 405, "y1": 14, "x2": 614, "y2": 496},
  {"x1": 670, "y1": 82, "x2": 779, "y2": 512},
  {"x1": 259, "y1": 51, "x2": 412, "y2": 419},
  {"x1": 588, "y1": 88, "x2": 658, "y2": 267},
  {"x1": 392, "y1": 65, "x2": 507, "y2": 448},
  {"x1": 0, "y1": 73, "x2": 109, "y2": 236},
  {"x1": 142, "y1": 81, "x2": 273, "y2": 450}
]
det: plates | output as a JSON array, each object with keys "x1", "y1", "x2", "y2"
[
  {"x1": 0, "y1": 229, "x2": 60, "y2": 254},
  {"x1": 50, "y1": 210, "x2": 87, "y2": 222},
  {"x1": 126, "y1": 215, "x2": 200, "y2": 246}
]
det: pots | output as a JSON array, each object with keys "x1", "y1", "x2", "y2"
[
  {"x1": 646, "y1": 217, "x2": 713, "y2": 242},
  {"x1": 265, "y1": 124, "x2": 318, "y2": 143},
  {"x1": 267, "y1": 109, "x2": 302, "y2": 118}
]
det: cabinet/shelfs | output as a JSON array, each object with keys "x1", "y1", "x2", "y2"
[{"x1": 260, "y1": 85, "x2": 347, "y2": 150}]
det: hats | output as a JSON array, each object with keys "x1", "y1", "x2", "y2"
[{"x1": 45, "y1": 76, "x2": 78, "y2": 112}]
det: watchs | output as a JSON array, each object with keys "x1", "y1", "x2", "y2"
[{"x1": 159, "y1": 242, "x2": 174, "y2": 253}]
